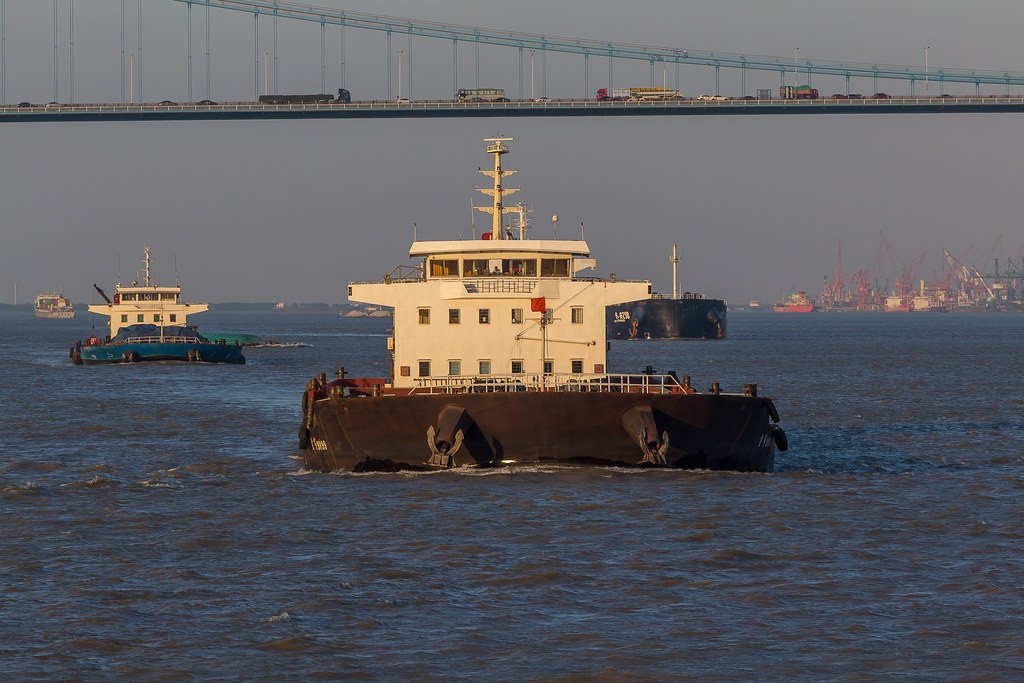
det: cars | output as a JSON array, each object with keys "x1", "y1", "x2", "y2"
[
  {"x1": 697, "y1": 94, "x2": 710, "y2": 101},
  {"x1": 158, "y1": 101, "x2": 178, "y2": 106},
  {"x1": 494, "y1": 97, "x2": 510, "y2": 102},
  {"x1": 849, "y1": 94, "x2": 859, "y2": 99},
  {"x1": 710, "y1": 94, "x2": 728, "y2": 100},
  {"x1": 46, "y1": 102, "x2": 62, "y2": 108},
  {"x1": 195, "y1": 100, "x2": 218, "y2": 105},
  {"x1": 17, "y1": 102, "x2": 38, "y2": 107},
  {"x1": 744, "y1": 96, "x2": 755, "y2": 100},
  {"x1": 873, "y1": 93, "x2": 891, "y2": 99},
  {"x1": 831, "y1": 94, "x2": 847, "y2": 99},
  {"x1": 536, "y1": 96, "x2": 553, "y2": 102},
  {"x1": 937, "y1": 94, "x2": 953, "y2": 98},
  {"x1": 671, "y1": 96, "x2": 686, "y2": 101}
]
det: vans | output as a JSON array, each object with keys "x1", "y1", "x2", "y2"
[{"x1": 397, "y1": 98, "x2": 410, "y2": 102}]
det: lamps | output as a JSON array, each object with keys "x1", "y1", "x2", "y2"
[{"x1": 609, "y1": 272, "x2": 617, "y2": 284}]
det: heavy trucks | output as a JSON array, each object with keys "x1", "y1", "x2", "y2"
[
  {"x1": 780, "y1": 85, "x2": 819, "y2": 99},
  {"x1": 259, "y1": 88, "x2": 351, "y2": 105},
  {"x1": 595, "y1": 88, "x2": 680, "y2": 101}
]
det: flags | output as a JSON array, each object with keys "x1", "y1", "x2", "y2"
[{"x1": 531, "y1": 297, "x2": 545, "y2": 312}]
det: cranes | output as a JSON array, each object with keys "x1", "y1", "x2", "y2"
[{"x1": 821, "y1": 229, "x2": 980, "y2": 312}]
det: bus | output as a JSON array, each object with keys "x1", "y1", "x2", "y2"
[{"x1": 457, "y1": 88, "x2": 505, "y2": 103}]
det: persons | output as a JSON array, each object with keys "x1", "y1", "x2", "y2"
[{"x1": 474, "y1": 263, "x2": 518, "y2": 277}]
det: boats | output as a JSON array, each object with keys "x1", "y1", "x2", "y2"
[
  {"x1": 750, "y1": 298, "x2": 760, "y2": 307},
  {"x1": 33, "y1": 289, "x2": 76, "y2": 319},
  {"x1": 606, "y1": 243, "x2": 728, "y2": 341},
  {"x1": 772, "y1": 292, "x2": 815, "y2": 313},
  {"x1": 298, "y1": 132, "x2": 788, "y2": 473},
  {"x1": 70, "y1": 244, "x2": 246, "y2": 365}
]
